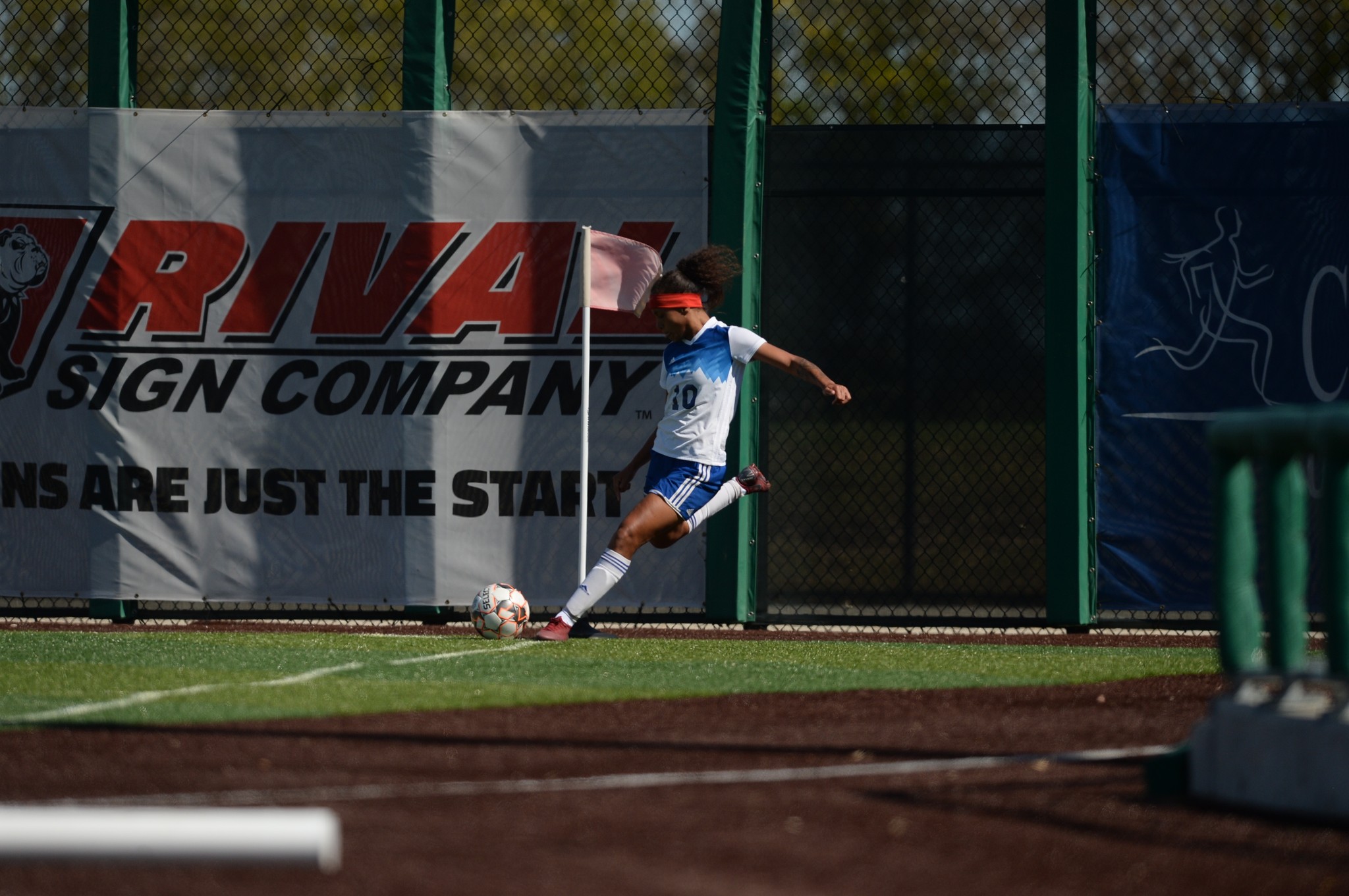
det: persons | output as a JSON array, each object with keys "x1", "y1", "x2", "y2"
[{"x1": 532, "y1": 244, "x2": 850, "y2": 639}]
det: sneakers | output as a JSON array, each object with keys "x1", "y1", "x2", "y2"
[
  {"x1": 736, "y1": 464, "x2": 771, "y2": 495},
  {"x1": 536, "y1": 617, "x2": 572, "y2": 640}
]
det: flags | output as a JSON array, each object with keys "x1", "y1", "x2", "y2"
[{"x1": 590, "y1": 231, "x2": 663, "y2": 319}]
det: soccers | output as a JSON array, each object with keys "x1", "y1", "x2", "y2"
[{"x1": 470, "y1": 582, "x2": 530, "y2": 640}]
